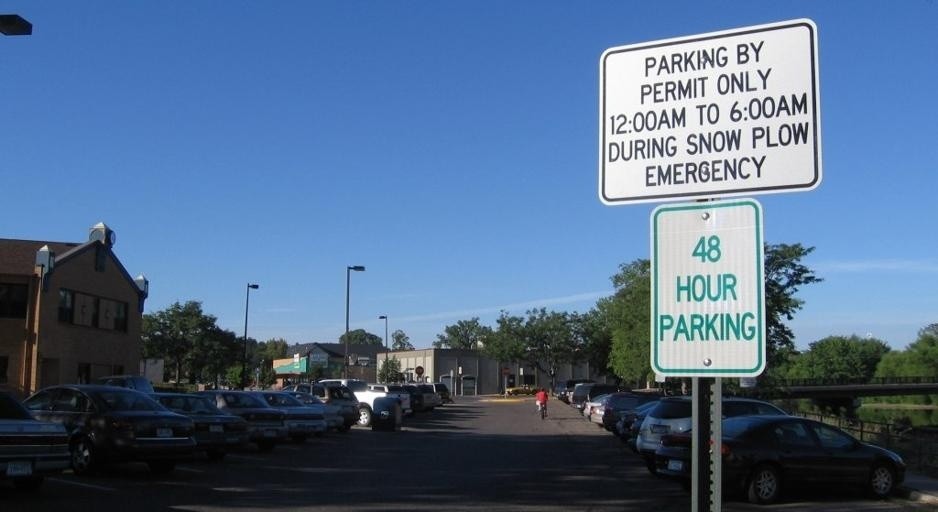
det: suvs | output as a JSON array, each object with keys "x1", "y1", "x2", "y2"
[
  {"x1": 635, "y1": 394, "x2": 788, "y2": 477},
  {"x1": 283, "y1": 382, "x2": 362, "y2": 432}
]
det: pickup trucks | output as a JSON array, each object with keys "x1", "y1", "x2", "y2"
[{"x1": 318, "y1": 379, "x2": 413, "y2": 427}]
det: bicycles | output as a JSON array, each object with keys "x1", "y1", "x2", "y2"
[{"x1": 535, "y1": 399, "x2": 548, "y2": 420}]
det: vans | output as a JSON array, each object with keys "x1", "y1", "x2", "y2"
[
  {"x1": 97, "y1": 375, "x2": 154, "y2": 393},
  {"x1": 368, "y1": 383, "x2": 451, "y2": 417}
]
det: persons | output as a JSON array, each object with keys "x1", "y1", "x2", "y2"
[{"x1": 536, "y1": 387, "x2": 548, "y2": 417}]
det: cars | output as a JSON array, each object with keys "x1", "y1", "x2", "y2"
[
  {"x1": 199, "y1": 389, "x2": 289, "y2": 450},
  {"x1": 144, "y1": 392, "x2": 248, "y2": 457},
  {"x1": 245, "y1": 391, "x2": 329, "y2": 445},
  {"x1": 654, "y1": 415, "x2": 908, "y2": 503},
  {"x1": 558, "y1": 379, "x2": 659, "y2": 454},
  {"x1": 1, "y1": 392, "x2": 72, "y2": 499},
  {"x1": 506, "y1": 384, "x2": 545, "y2": 396},
  {"x1": 22, "y1": 384, "x2": 194, "y2": 477},
  {"x1": 281, "y1": 391, "x2": 344, "y2": 437}
]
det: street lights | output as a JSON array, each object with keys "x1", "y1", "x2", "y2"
[
  {"x1": 241, "y1": 282, "x2": 260, "y2": 391},
  {"x1": 378, "y1": 315, "x2": 390, "y2": 384},
  {"x1": 344, "y1": 265, "x2": 365, "y2": 378}
]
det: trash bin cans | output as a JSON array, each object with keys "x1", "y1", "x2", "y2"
[{"x1": 371, "y1": 397, "x2": 402, "y2": 432}]
